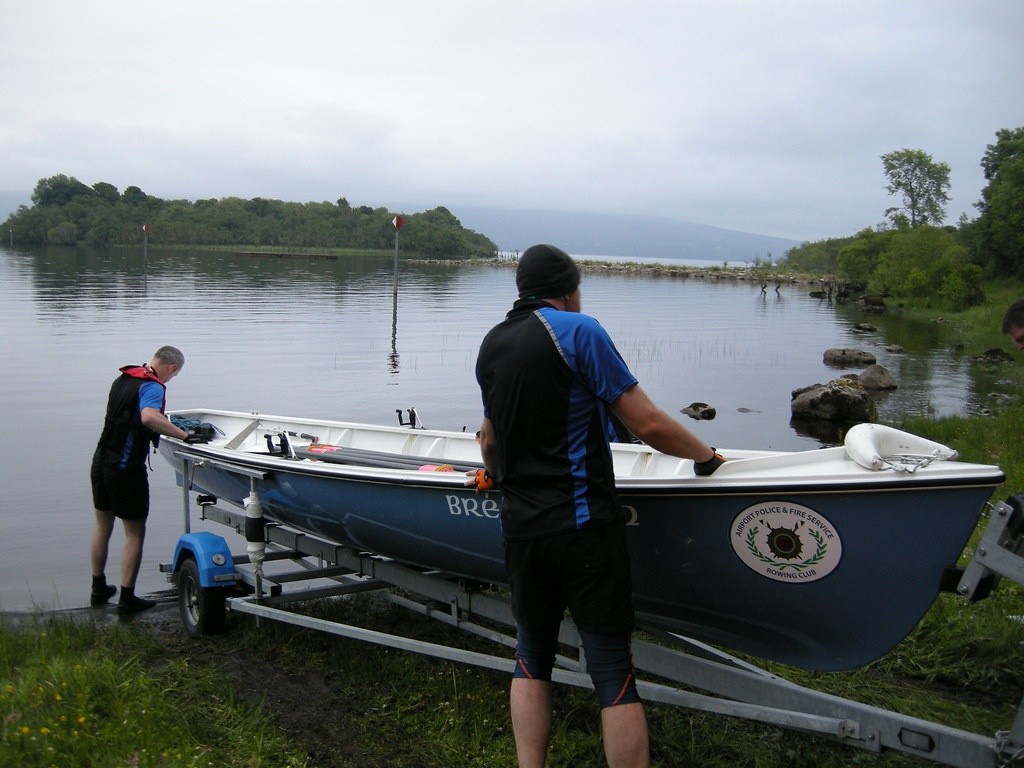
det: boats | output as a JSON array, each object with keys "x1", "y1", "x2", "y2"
[{"x1": 150, "y1": 407, "x2": 1009, "y2": 672}]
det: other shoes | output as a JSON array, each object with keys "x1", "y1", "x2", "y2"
[
  {"x1": 116, "y1": 599, "x2": 156, "y2": 616},
  {"x1": 91, "y1": 584, "x2": 117, "y2": 606}
]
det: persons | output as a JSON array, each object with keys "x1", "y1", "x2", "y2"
[
  {"x1": 462, "y1": 245, "x2": 727, "y2": 768},
  {"x1": 1002, "y1": 300, "x2": 1024, "y2": 351},
  {"x1": 89, "y1": 346, "x2": 210, "y2": 619}
]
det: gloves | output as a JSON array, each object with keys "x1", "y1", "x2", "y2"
[{"x1": 182, "y1": 431, "x2": 208, "y2": 444}]
